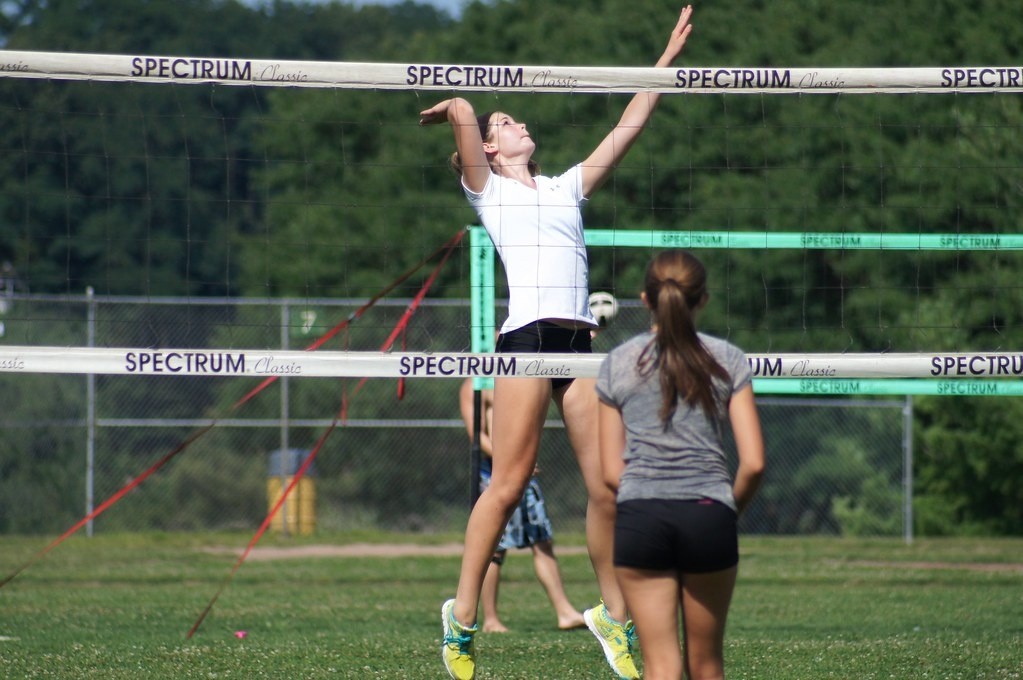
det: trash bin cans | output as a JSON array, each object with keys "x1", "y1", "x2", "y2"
[{"x1": 269, "y1": 448, "x2": 316, "y2": 535}]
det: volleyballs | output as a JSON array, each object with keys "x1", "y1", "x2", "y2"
[{"x1": 586, "y1": 291, "x2": 619, "y2": 324}]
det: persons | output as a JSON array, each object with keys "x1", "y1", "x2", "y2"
[
  {"x1": 417, "y1": 4, "x2": 696, "y2": 680},
  {"x1": 594, "y1": 247, "x2": 765, "y2": 679},
  {"x1": 458, "y1": 327, "x2": 599, "y2": 632}
]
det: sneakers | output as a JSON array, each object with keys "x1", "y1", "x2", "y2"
[
  {"x1": 441, "y1": 599, "x2": 477, "y2": 680},
  {"x1": 583, "y1": 597, "x2": 640, "y2": 679}
]
